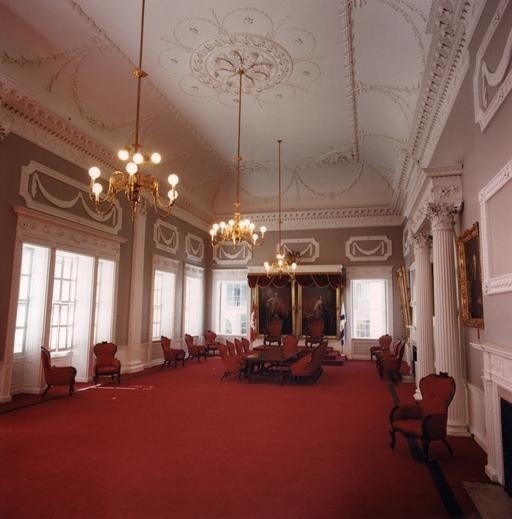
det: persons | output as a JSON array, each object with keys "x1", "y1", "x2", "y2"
[
  {"x1": 306, "y1": 294, "x2": 328, "y2": 320},
  {"x1": 266, "y1": 292, "x2": 283, "y2": 320},
  {"x1": 468, "y1": 250, "x2": 483, "y2": 319}
]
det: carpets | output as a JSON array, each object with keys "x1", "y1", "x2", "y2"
[{"x1": 461, "y1": 479, "x2": 512, "y2": 519}]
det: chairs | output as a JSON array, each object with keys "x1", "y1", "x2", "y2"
[
  {"x1": 370, "y1": 334, "x2": 406, "y2": 381},
  {"x1": 389, "y1": 372, "x2": 455, "y2": 463},
  {"x1": 41, "y1": 341, "x2": 121, "y2": 396},
  {"x1": 162, "y1": 314, "x2": 330, "y2": 385}
]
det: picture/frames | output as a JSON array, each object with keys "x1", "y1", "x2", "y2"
[
  {"x1": 256, "y1": 280, "x2": 340, "y2": 340},
  {"x1": 454, "y1": 222, "x2": 485, "y2": 328}
]
